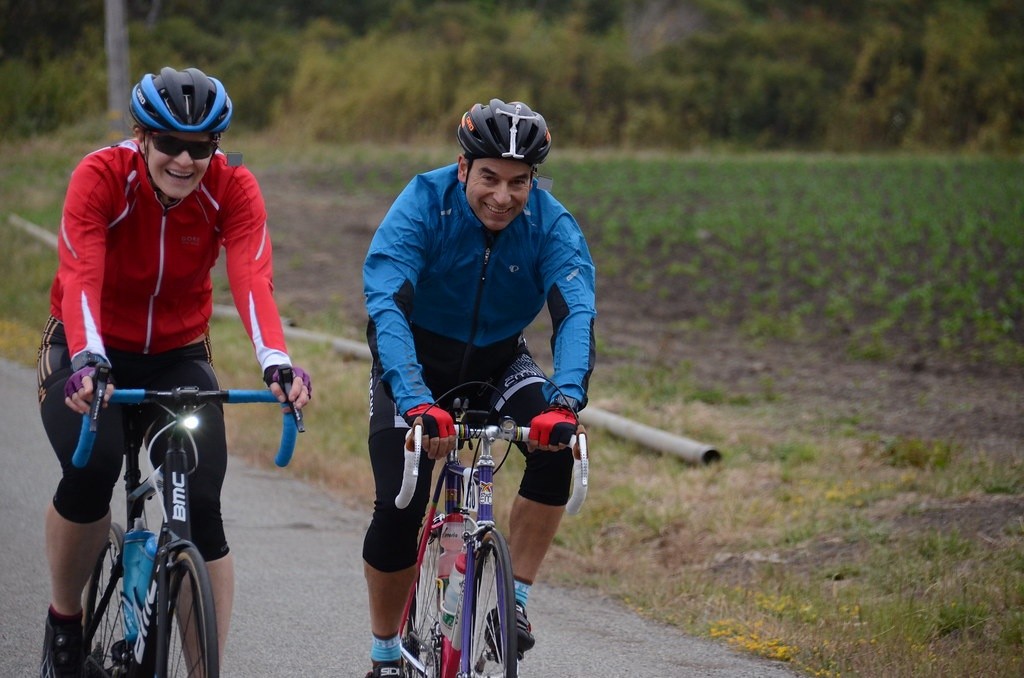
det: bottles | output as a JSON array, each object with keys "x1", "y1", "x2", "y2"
[
  {"x1": 439, "y1": 553, "x2": 469, "y2": 639},
  {"x1": 132, "y1": 535, "x2": 164, "y2": 634},
  {"x1": 120, "y1": 516, "x2": 154, "y2": 641},
  {"x1": 435, "y1": 507, "x2": 466, "y2": 615}
]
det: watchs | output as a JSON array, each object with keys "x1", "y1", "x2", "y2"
[
  {"x1": 71, "y1": 350, "x2": 106, "y2": 372},
  {"x1": 549, "y1": 394, "x2": 580, "y2": 417}
]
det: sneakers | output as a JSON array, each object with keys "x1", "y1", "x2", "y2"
[
  {"x1": 39, "y1": 617, "x2": 85, "y2": 678},
  {"x1": 486, "y1": 608, "x2": 534, "y2": 651},
  {"x1": 367, "y1": 658, "x2": 405, "y2": 678}
]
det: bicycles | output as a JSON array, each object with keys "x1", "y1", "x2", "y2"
[
  {"x1": 395, "y1": 418, "x2": 589, "y2": 677},
  {"x1": 71, "y1": 364, "x2": 306, "y2": 678}
]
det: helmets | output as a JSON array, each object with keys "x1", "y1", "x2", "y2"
[
  {"x1": 130, "y1": 68, "x2": 234, "y2": 134},
  {"x1": 456, "y1": 98, "x2": 553, "y2": 166}
]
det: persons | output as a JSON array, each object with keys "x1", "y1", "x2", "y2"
[
  {"x1": 35, "y1": 64, "x2": 312, "y2": 677},
  {"x1": 362, "y1": 98, "x2": 598, "y2": 678}
]
